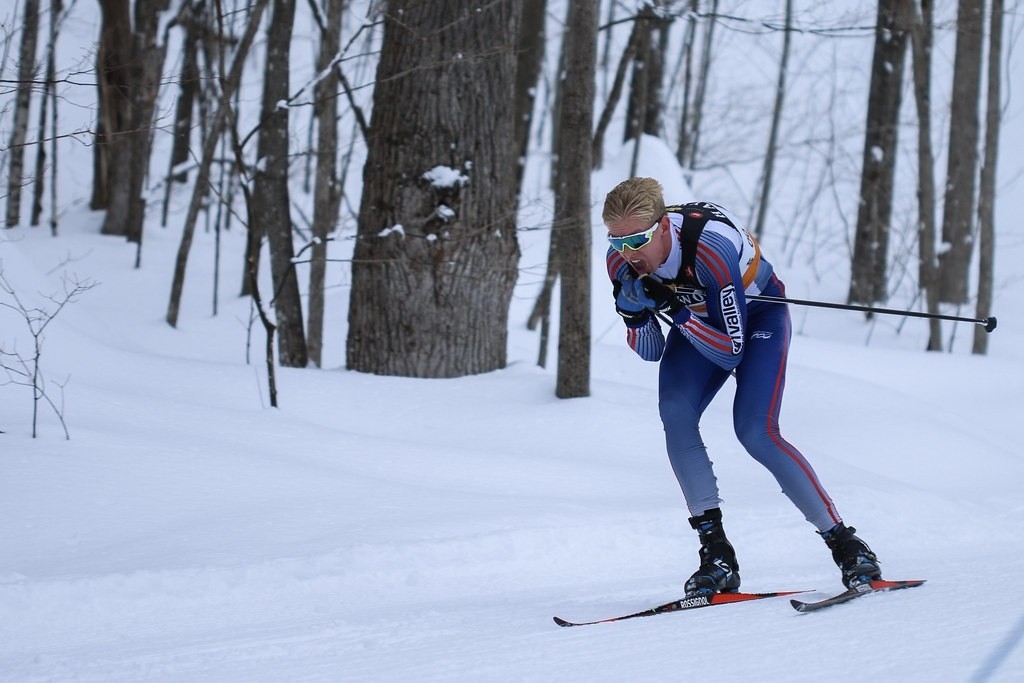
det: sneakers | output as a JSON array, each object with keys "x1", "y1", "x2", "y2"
[
  {"x1": 821, "y1": 523, "x2": 882, "y2": 589},
  {"x1": 685, "y1": 524, "x2": 741, "y2": 595}
]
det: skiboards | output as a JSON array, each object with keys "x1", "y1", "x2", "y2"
[{"x1": 552, "y1": 576, "x2": 928, "y2": 632}]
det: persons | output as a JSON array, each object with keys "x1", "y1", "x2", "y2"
[{"x1": 602, "y1": 176, "x2": 882, "y2": 593}]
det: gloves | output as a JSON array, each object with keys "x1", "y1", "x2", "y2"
[
  {"x1": 613, "y1": 281, "x2": 649, "y2": 325},
  {"x1": 623, "y1": 274, "x2": 686, "y2": 316}
]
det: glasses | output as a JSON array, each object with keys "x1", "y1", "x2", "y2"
[{"x1": 606, "y1": 214, "x2": 664, "y2": 252}]
